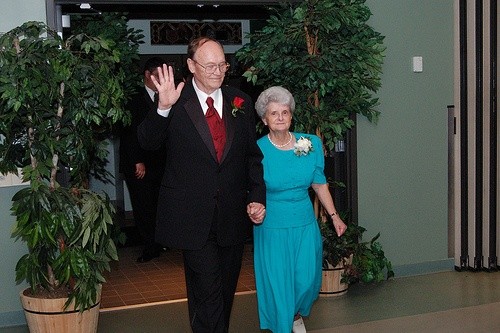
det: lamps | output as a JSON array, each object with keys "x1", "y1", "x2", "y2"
[{"x1": 79, "y1": 3, "x2": 91, "y2": 9}]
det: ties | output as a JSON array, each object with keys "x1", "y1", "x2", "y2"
[
  {"x1": 205, "y1": 96, "x2": 225, "y2": 163},
  {"x1": 154, "y1": 92, "x2": 159, "y2": 103}
]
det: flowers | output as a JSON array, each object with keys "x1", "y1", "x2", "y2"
[
  {"x1": 293, "y1": 136, "x2": 315, "y2": 157},
  {"x1": 231, "y1": 97, "x2": 246, "y2": 117}
]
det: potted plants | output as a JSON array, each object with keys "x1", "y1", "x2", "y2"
[
  {"x1": 0, "y1": 14, "x2": 143, "y2": 333},
  {"x1": 234, "y1": 0, "x2": 387, "y2": 299}
]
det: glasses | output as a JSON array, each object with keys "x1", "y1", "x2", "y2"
[{"x1": 192, "y1": 60, "x2": 230, "y2": 74}]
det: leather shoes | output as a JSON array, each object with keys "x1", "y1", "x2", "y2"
[
  {"x1": 137, "y1": 248, "x2": 159, "y2": 263},
  {"x1": 158, "y1": 243, "x2": 169, "y2": 252}
]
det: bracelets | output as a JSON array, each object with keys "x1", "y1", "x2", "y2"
[{"x1": 331, "y1": 212, "x2": 338, "y2": 218}]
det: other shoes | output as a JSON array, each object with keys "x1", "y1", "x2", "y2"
[{"x1": 293, "y1": 317, "x2": 306, "y2": 333}]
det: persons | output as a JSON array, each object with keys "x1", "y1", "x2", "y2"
[
  {"x1": 248, "y1": 87, "x2": 348, "y2": 333},
  {"x1": 119, "y1": 56, "x2": 166, "y2": 263},
  {"x1": 136, "y1": 36, "x2": 266, "y2": 333}
]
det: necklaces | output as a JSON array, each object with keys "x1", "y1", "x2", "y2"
[{"x1": 267, "y1": 132, "x2": 292, "y2": 147}]
